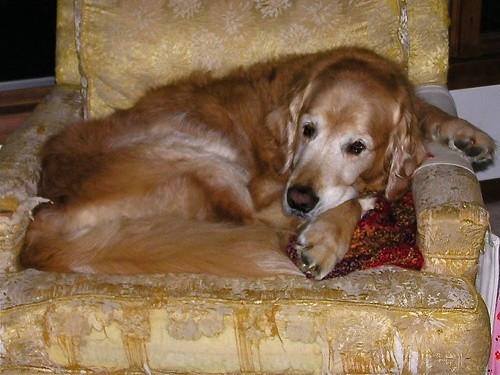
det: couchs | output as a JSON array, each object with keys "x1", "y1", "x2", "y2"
[{"x1": 0, "y1": 0, "x2": 492, "y2": 375}]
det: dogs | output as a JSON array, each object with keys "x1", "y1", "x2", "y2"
[{"x1": 19, "y1": 45, "x2": 498, "y2": 283}]
